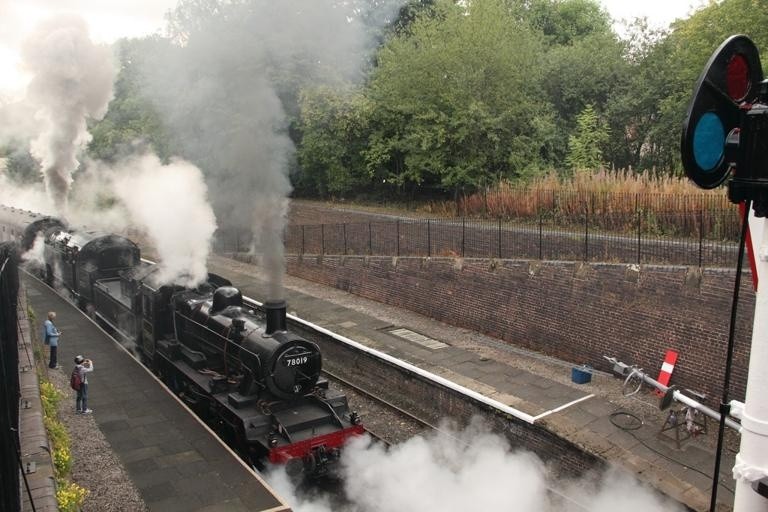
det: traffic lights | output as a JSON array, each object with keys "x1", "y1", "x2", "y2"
[{"x1": 682, "y1": 33, "x2": 768, "y2": 217}]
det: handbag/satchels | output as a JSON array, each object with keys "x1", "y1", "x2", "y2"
[
  {"x1": 43, "y1": 325, "x2": 50, "y2": 345},
  {"x1": 70, "y1": 365, "x2": 82, "y2": 391}
]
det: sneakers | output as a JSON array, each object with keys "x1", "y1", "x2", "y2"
[
  {"x1": 49, "y1": 362, "x2": 59, "y2": 370},
  {"x1": 77, "y1": 407, "x2": 94, "y2": 414}
]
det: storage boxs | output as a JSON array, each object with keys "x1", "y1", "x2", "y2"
[{"x1": 571, "y1": 365, "x2": 592, "y2": 384}]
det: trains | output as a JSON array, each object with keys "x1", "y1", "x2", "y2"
[{"x1": 0, "y1": 203, "x2": 366, "y2": 475}]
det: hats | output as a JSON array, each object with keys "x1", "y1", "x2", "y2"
[{"x1": 74, "y1": 354, "x2": 85, "y2": 363}]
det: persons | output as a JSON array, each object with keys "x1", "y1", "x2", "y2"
[
  {"x1": 71, "y1": 353, "x2": 95, "y2": 415},
  {"x1": 43, "y1": 309, "x2": 63, "y2": 370}
]
